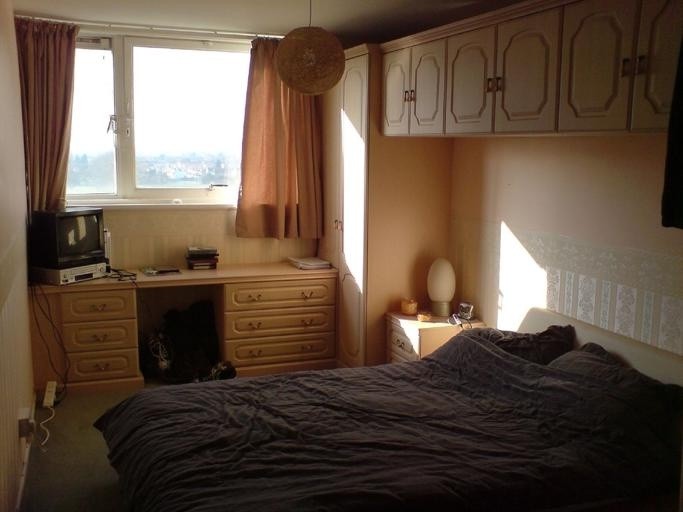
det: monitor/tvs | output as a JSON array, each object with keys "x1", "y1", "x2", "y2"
[{"x1": 31, "y1": 207, "x2": 106, "y2": 269}]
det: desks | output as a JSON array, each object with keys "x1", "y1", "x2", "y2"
[{"x1": 33, "y1": 262, "x2": 340, "y2": 394}]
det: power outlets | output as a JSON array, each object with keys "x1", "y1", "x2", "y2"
[{"x1": 19, "y1": 408, "x2": 37, "y2": 438}]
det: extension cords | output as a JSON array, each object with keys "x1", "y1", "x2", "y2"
[{"x1": 43, "y1": 381, "x2": 57, "y2": 408}]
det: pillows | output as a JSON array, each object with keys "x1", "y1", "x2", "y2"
[
  {"x1": 541, "y1": 348, "x2": 683, "y2": 404},
  {"x1": 488, "y1": 326, "x2": 578, "y2": 361}
]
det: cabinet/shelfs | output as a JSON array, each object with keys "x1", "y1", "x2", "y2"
[
  {"x1": 382, "y1": 26, "x2": 446, "y2": 140},
  {"x1": 215, "y1": 283, "x2": 340, "y2": 377},
  {"x1": 449, "y1": 0, "x2": 560, "y2": 138},
  {"x1": 561, "y1": 3, "x2": 681, "y2": 131},
  {"x1": 317, "y1": 52, "x2": 453, "y2": 369},
  {"x1": 55, "y1": 288, "x2": 144, "y2": 400}
]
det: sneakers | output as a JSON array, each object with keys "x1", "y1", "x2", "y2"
[
  {"x1": 191, "y1": 359, "x2": 239, "y2": 384},
  {"x1": 147, "y1": 330, "x2": 177, "y2": 377}
]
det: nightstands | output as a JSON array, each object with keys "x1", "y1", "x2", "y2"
[{"x1": 386, "y1": 296, "x2": 473, "y2": 361}]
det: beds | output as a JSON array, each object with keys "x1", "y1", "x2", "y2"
[{"x1": 94, "y1": 325, "x2": 683, "y2": 510}]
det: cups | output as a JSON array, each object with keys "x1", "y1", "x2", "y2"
[
  {"x1": 400, "y1": 298, "x2": 417, "y2": 316},
  {"x1": 416, "y1": 312, "x2": 432, "y2": 322}
]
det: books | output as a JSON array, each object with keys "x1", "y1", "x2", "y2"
[{"x1": 289, "y1": 257, "x2": 330, "y2": 270}]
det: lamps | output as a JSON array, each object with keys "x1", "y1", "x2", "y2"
[
  {"x1": 275, "y1": 0, "x2": 350, "y2": 97},
  {"x1": 426, "y1": 255, "x2": 459, "y2": 317}
]
